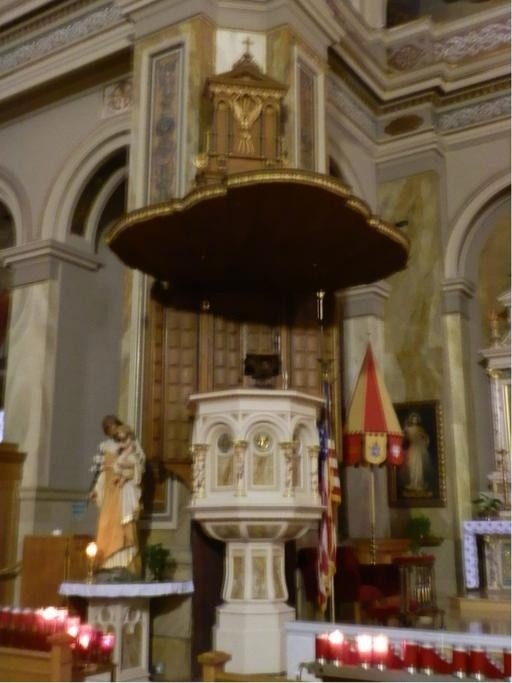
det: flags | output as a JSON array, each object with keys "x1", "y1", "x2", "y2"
[{"x1": 315, "y1": 382, "x2": 342, "y2": 616}]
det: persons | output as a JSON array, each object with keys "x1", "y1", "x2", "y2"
[
  {"x1": 92, "y1": 415, "x2": 143, "y2": 577},
  {"x1": 406, "y1": 412, "x2": 431, "y2": 490},
  {"x1": 113, "y1": 424, "x2": 137, "y2": 486}
]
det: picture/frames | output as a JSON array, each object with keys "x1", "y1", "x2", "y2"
[{"x1": 387, "y1": 400, "x2": 447, "y2": 507}]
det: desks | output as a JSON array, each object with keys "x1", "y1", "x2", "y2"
[{"x1": 283, "y1": 618, "x2": 510, "y2": 681}]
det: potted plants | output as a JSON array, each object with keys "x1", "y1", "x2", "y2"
[
  {"x1": 472, "y1": 493, "x2": 502, "y2": 521},
  {"x1": 145, "y1": 542, "x2": 170, "y2": 583}
]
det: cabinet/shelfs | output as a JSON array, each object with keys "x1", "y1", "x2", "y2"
[{"x1": 19, "y1": 533, "x2": 91, "y2": 622}]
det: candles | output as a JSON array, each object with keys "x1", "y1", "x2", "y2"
[
  {"x1": 315, "y1": 629, "x2": 511, "y2": 681},
  {"x1": 0, "y1": 605, "x2": 116, "y2": 661}
]
